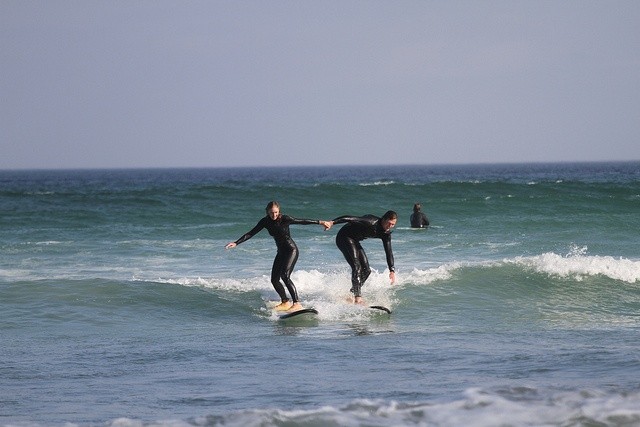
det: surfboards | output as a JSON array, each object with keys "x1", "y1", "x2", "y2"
[
  {"x1": 345, "y1": 298, "x2": 392, "y2": 314},
  {"x1": 265, "y1": 301, "x2": 318, "y2": 321}
]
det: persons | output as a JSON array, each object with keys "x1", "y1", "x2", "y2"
[
  {"x1": 224, "y1": 200, "x2": 328, "y2": 312},
  {"x1": 325, "y1": 210, "x2": 400, "y2": 307},
  {"x1": 410, "y1": 199, "x2": 429, "y2": 231}
]
